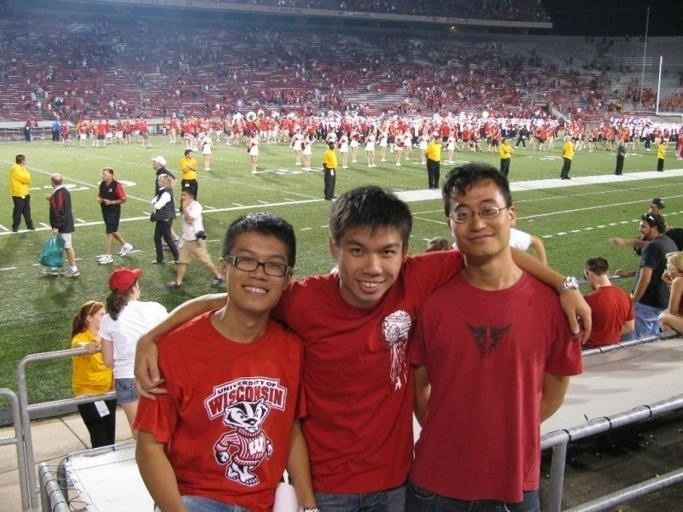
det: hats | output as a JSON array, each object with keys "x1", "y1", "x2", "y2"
[
  {"x1": 151, "y1": 156, "x2": 166, "y2": 166},
  {"x1": 653, "y1": 198, "x2": 665, "y2": 208},
  {"x1": 109, "y1": 267, "x2": 142, "y2": 291}
]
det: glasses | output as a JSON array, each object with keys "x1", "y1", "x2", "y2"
[
  {"x1": 448, "y1": 207, "x2": 507, "y2": 223},
  {"x1": 225, "y1": 255, "x2": 292, "y2": 277}
]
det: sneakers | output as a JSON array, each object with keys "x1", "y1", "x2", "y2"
[
  {"x1": 119, "y1": 244, "x2": 133, "y2": 256},
  {"x1": 166, "y1": 280, "x2": 184, "y2": 289},
  {"x1": 97, "y1": 255, "x2": 113, "y2": 264},
  {"x1": 211, "y1": 276, "x2": 224, "y2": 288},
  {"x1": 61, "y1": 268, "x2": 80, "y2": 277},
  {"x1": 40, "y1": 267, "x2": 59, "y2": 276}
]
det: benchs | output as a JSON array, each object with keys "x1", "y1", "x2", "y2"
[{"x1": 1, "y1": 0, "x2": 683, "y2": 122}]
